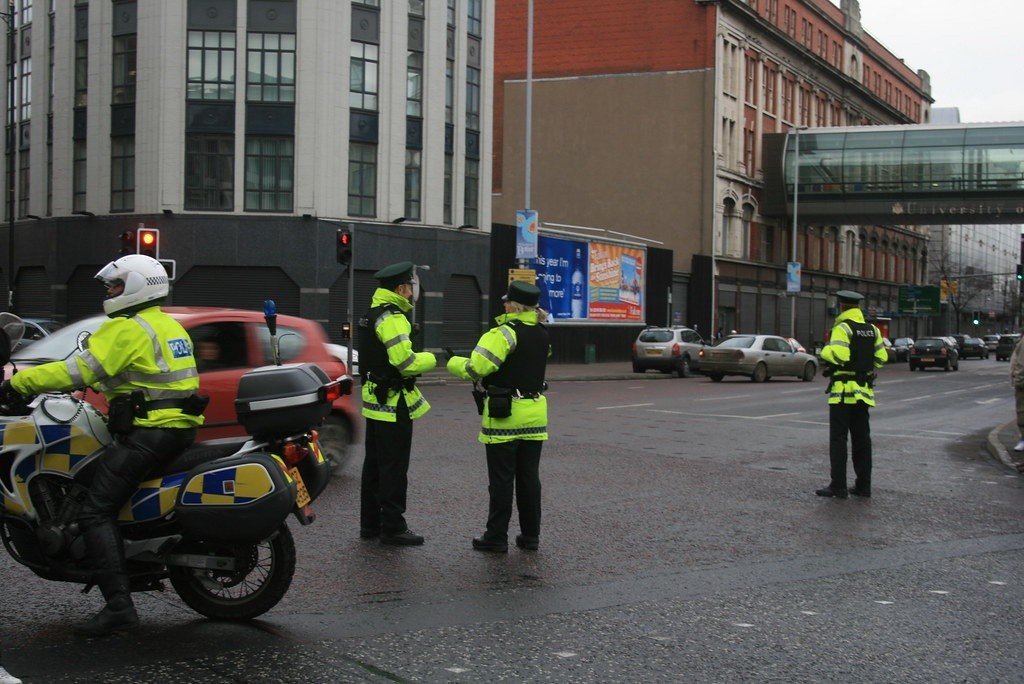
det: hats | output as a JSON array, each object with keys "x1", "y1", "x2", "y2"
[
  {"x1": 836, "y1": 290, "x2": 865, "y2": 305},
  {"x1": 376, "y1": 262, "x2": 417, "y2": 285},
  {"x1": 501, "y1": 280, "x2": 540, "y2": 306}
]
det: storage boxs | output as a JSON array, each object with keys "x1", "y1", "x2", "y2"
[{"x1": 234, "y1": 362, "x2": 333, "y2": 439}]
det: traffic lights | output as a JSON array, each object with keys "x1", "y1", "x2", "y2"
[
  {"x1": 336, "y1": 228, "x2": 352, "y2": 265},
  {"x1": 117, "y1": 227, "x2": 136, "y2": 258},
  {"x1": 1016, "y1": 264, "x2": 1023, "y2": 280},
  {"x1": 137, "y1": 228, "x2": 176, "y2": 281},
  {"x1": 972, "y1": 310, "x2": 980, "y2": 326}
]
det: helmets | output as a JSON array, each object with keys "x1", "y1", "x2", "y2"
[{"x1": 99, "y1": 254, "x2": 169, "y2": 317}]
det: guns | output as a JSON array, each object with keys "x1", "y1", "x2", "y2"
[{"x1": 366, "y1": 371, "x2": 388, "y2": 405}]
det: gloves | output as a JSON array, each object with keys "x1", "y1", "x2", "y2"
[{"x1": 0, "y1": 380, "x2": 20, "y2": 406}]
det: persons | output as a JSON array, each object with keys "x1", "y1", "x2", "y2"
[
  {"x1": 358, "y1": 261, "x2": 437, "y2": 546},
  {"x1": 1010, "y1": 318, "x2": 1024, "y2": 451},
  {"x1": 693, "y1": 324, "x2": 737, "y2": 345},
  {"x1": 815, "y1": 290, "x2": 888, "y2": 499},
  {"x1": 445, "y1": 281, "x2": 552, "y2": 552},
  {"x1": 0, "y1": 254, "x2": 206, "y2": 635}
]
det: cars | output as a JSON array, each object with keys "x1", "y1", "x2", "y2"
[
  {"x1": 982, "y1": 335, "x2": 1000, "y2": 352},
  {"x1": 3, "y1": 319, "x2": 67, "y2": 355},
  {"x1": 4, "y1": 306, "x2": 360, "y2": 472},
  {"x1": 696, "y1": 334, "x2": 820, "y2": 383},
  {"x1": 946, "y1": 335, "x2": 990, "y2": 360},
  {"x1": 909, "y1": 337, "x2": 960, "y2": 371},
  {"x1": 996, "y1": 333, "x2": 1021, "y2": 361},
  {"x1": 324, "y1": 343, "x2": 360, "y2": 376},
  {"x1": 882, "y1": 337, "x2": 898, "y2": 361},
  {"x1": 893, "y1": 337, "x2": 914, "y2": 361}
]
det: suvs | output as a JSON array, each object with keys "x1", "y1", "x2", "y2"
[{"x1": 632, "y1": 324, "x2": 707, "y2": 378}]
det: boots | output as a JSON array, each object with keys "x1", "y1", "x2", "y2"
[{"x1": 75, "y1": 524, "x2": 141, "y2": 637}]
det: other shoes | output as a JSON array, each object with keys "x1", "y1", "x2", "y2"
[
  {"x1": 849, "y1": 486, "x2": 870, "y2": 497},
  {"x1": 816, "y1": 485, "x2": 848, "y2": 499}
]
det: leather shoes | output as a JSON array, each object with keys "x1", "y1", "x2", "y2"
[
  {"x1": 379, "y1": 529, "x2": 423, "y2": 544},
  {"x1": 359, "y1": 526, "x2": 380, "y2": 539}
]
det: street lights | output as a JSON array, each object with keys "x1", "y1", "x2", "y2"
[
  {"x1": 791, "y1": 126, "x2": 807, "y2": 338},
  {"x1": 412, "y1": 265, "x2": 430, "y2": 377}
]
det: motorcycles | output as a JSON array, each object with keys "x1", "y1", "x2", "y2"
[{"x1": 0, "y1": 299, "x2": 342, "y2": 623}]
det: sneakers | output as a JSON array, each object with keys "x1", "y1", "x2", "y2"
[
  {"x1": 516, "y1": 533, "x2": 539, "y2": 552},
  {"x1": 473, "y1": 534, "x2": 508, "y2": 552}
]
